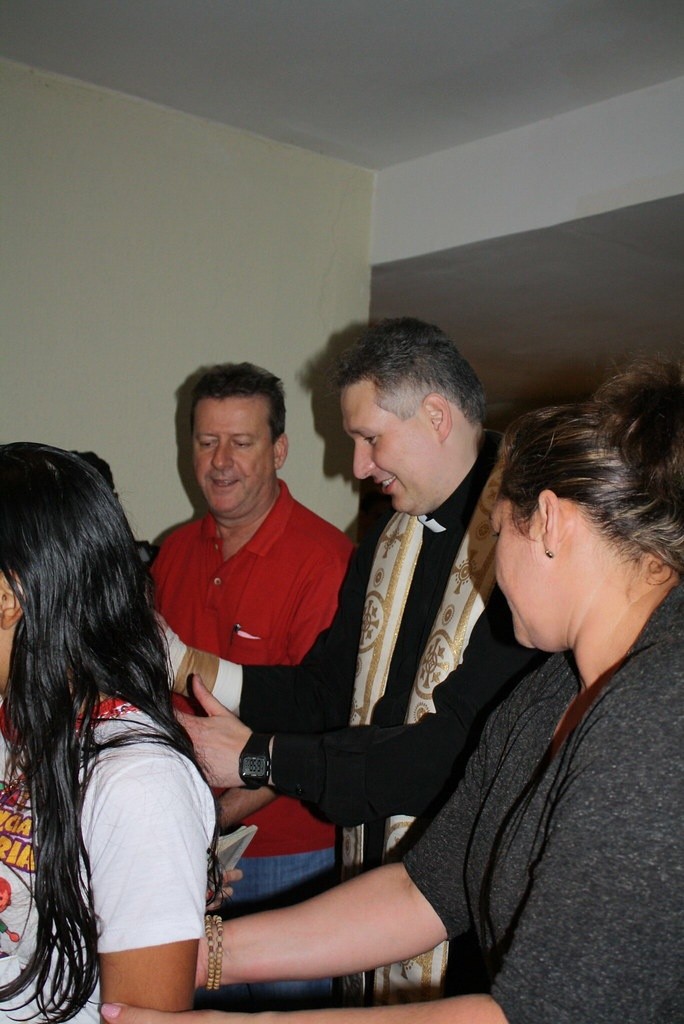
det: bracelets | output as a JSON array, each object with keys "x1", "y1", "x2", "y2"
[{"x1": 205, "y1": 915, "x2": 222, "y2": 991}]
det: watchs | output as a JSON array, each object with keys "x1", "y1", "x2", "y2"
[{"x1": 239, "y1": 730, "x2": 275, "y2": 789}]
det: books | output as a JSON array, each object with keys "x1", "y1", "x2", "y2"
[{"x1": 206, "y1": 826, "x2": 257, "y2": 906}]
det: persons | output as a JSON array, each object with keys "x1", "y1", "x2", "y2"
[
  {"x1": 100, "y1": 365, "x2": 684, "y2": 1024},
  {"x1": 74, "y1": 451, "x2": 241, "y2": 911},
  {"x1": 0, "y1": 441, "x2": 215, "y2": 1023},
  {"x1": 151, "y1": 361, "x2": 357, "y2": 1013},
  {"x1": 152, "y1": 318, "x2": 553, "y2": 1011}
]
